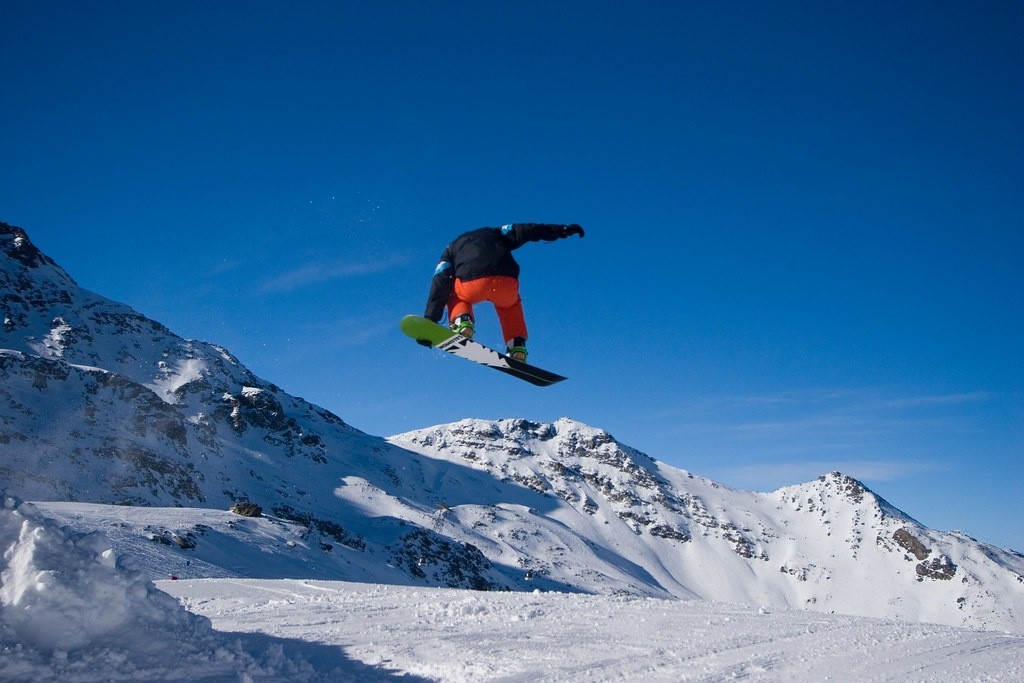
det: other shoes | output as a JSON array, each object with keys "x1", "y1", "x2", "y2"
[
  {"x1": 506, "y1": 337, "x2": 529, "y2": 364},
  {"x1": 449, "y1": 314, "x2": 475, "y2": 339}
]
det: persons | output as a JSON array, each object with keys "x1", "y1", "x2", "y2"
[{"x1": 415, "y1": 222, "x2": 585, "y2": 363}]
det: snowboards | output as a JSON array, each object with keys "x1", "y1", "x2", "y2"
[{"x1": 401, "y1": 313, "x2": 568, "y2": 386}]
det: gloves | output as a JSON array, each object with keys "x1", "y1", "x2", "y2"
[
  {"x1": 416, "y1": 316, "x2": 439, "y2": 350},
  {"x1": 561, "y1": 224, "x2": 585, "y2": 238}
]
